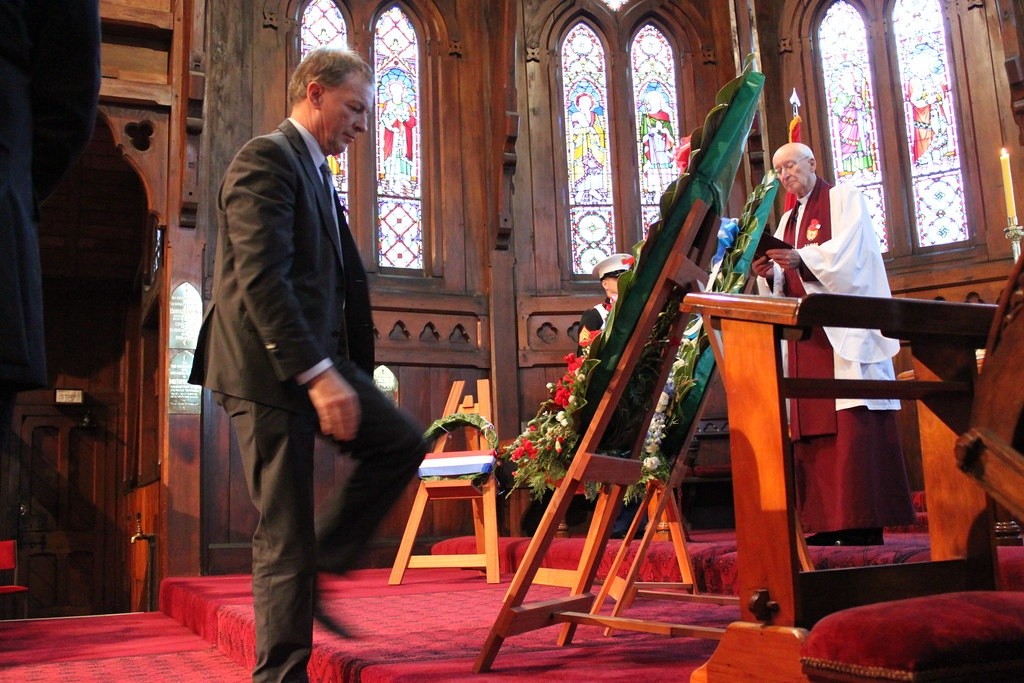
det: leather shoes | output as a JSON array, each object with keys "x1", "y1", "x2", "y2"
[{"x1": 611, "y1": 529, "x2": 644, "y2": 538}]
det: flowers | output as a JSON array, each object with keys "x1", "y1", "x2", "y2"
[{"x1": 500, "y1": 134, "x2": 739, "y2": 502}]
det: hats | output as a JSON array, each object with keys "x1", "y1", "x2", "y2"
[{"x1": 591, "y1": 253, "x2": 635, "y2": 281}]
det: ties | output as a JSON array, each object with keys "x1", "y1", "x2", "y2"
[{"x1": 320, "y1": 162, "x2": 346, "y2": 309}]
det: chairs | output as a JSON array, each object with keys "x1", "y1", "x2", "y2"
[{"x1": 0, "y1": 540, "x2": 29, "y2": 619}]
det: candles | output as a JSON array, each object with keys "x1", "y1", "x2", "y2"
[{"x1": 1000, "y1": 148, "x2": 1017, "y2": 217}]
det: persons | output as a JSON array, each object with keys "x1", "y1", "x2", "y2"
[
  {"x1": 578, "y1": 253, "x2": 650, "y2": 538},
  {"x1": 0, "y1": 0, "x2": 99, "y2": 388},
  {"x1": 753, "y1": 141, "x2": 903, "y2": 547},
  {"x1": 187, "y1": 47, "x2": 422, "y2": 683}
]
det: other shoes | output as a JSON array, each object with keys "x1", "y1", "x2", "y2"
[{"x1": 806, "y1": 530, "x2": 884, "y2": 547}]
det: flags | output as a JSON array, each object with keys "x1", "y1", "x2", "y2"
[{"x1": 783, "y1": 114, "x2": 806, "y2": 210}]
